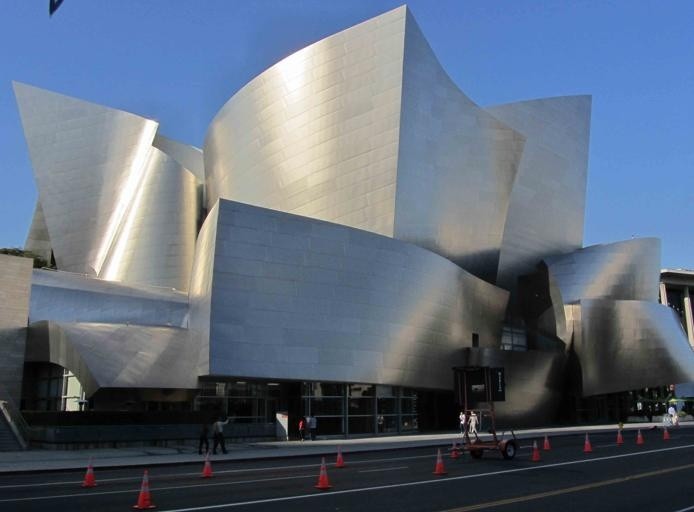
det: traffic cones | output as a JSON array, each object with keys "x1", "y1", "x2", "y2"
[
  {"x1": 199, "y1": 452, "x2": 216, "y2": 479},
  {"x1": 132, "y1": 468, "x2": 157, "y2": 509},
  {"x1": 635, "y1": 429, "x2": 645, "y2": 445},
  {"x1": 614, "y1": 429, "x2": 626, "y2": 444},
  {"x1": 662, "y1": 427, "x2": 671, "y2": 440},
  {"x1": 81, "y1": 454, "x2": 99, "y2": 489},
  {"x1": 431, "y1": 430, "x2": 594, "y2": 476},
  {"x1": 334, "y1": 445, "x2": 346, "y2": 468},
  {"x1": 313, "y1": 456, "x2": 334, "y2": 490}
]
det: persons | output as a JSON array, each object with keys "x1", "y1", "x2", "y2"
[
  {"x1": 667, "y1": 405, "x2": 675, "y2": 425},
  {"x1": 458, "y1": 411, "x2": 465, "y2": 434},
  {"x1": 466, "y1": 411, "x2": 479, "y2": 433},
  {"x1": 211, "y1": 416, "x2": 229, "y2": 454},
  {"x1": 376, "y1": 409, "x2": 384, "y2": 432},
  {"x1": 297, "y1": 415, "x2": 305, "y2": 441},
  {"x1": 198, "y1": 418, "x2": 209, "y2": 454},
  {"x1": 308, "y1": 413, "x2": 317, "y2": 440}
]
